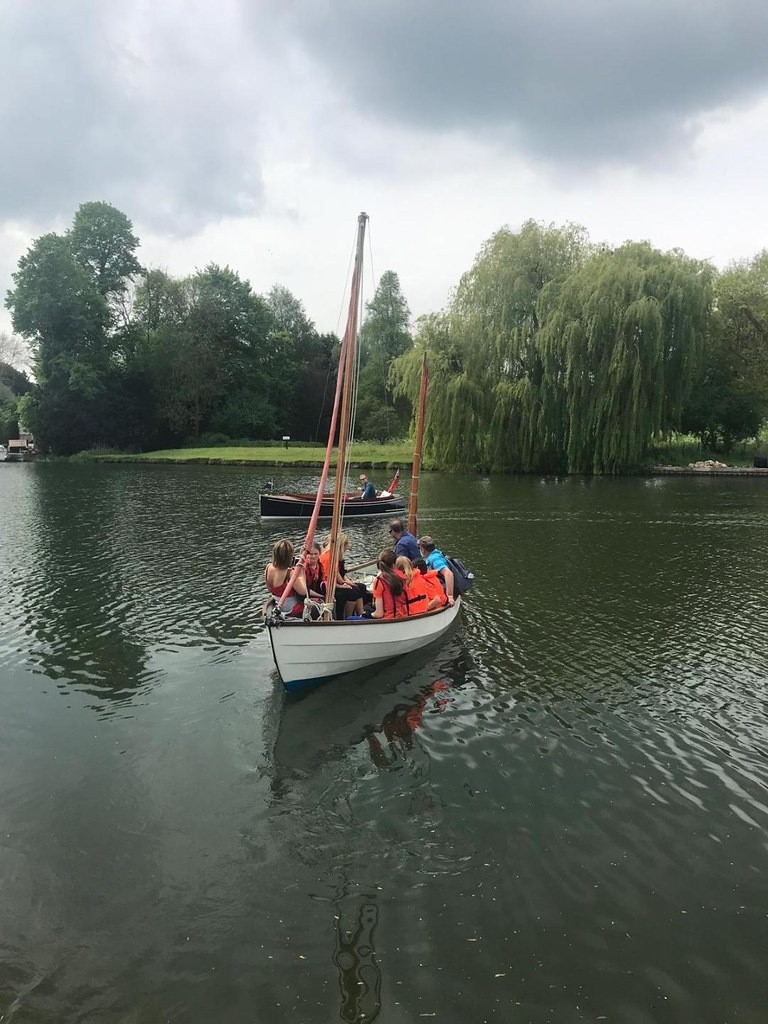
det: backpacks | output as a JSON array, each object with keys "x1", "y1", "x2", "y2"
[{"x1": 430, "y1": 548, "x2": 474, "y2": 595}]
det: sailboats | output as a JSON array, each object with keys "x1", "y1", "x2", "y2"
[{"x1": 263, "y1": 210, "x2": 462, "y2": 690}]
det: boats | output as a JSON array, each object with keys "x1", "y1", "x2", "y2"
[{"x1": 259, "y1": 488, "x2": 405, "y2": 520}]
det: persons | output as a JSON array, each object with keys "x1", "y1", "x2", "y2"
[
  {"x1": 346, "y1": 519, "x2": 455, "y2": 620},
  {"x1": 265, "y1": 533, "x2": 367, "y2": 620},
  {"x1": 357, "y1": 474, "x2": 376, "y2": 500}
]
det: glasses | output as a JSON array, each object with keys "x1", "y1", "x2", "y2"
[{"x1": 389, "y1": 530, "x2": 395, "y2": 534}]
